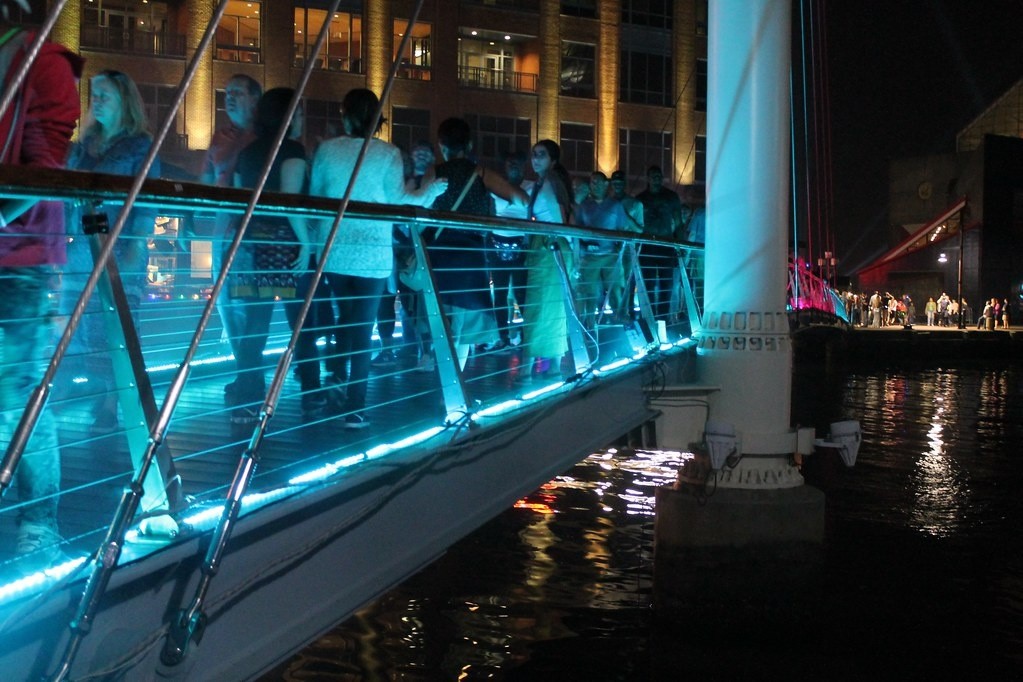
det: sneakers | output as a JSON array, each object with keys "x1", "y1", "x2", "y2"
[{"x1": 5, "y1": 520, "x2": 69, "y2": 575}]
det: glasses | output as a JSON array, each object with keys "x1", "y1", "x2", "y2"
[{"x1": 100, "y1": 68, "x2": 121, "y2": 77}]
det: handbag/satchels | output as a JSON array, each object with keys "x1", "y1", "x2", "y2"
[{"x1": 0, "y1": 200, "x2": 71, "y2": 263}]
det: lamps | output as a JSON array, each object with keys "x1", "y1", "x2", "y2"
[
  {"x1": 700, "y1": 418, "x2": 743, "y2": 472},
  {"x1": 813, "y1": 418, "x2": 863, "y2": 470}
]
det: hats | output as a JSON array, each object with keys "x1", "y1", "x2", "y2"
[{"x1": 610, "y1": 170, "x2": 625, "y2": 181}]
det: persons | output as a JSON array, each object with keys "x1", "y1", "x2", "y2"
[
  {"x1": 64, "y1": 68, "x2": 162, "y2": 435},
  {"x1": 176, "y1": 75, "x2": 705, "y2": 429},
  {"x1": 834, "y1": 288, "x2": 1010, "y2": 330},
  {"x1": 0, "y1": 0, "x2": 88, "y2": 576}
]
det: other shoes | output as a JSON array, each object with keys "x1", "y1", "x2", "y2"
[
  {"x1": 489, "y1": 338, "x2": 511, "y2": 351},
  {"x1": 87, "y1": 417, "x2": 120, "y2": 433},
  {"x1": 224, "y1": 373, "x2": 266, "y2": 393},
  {"x1": 229, "y1": 407, "x2": 261, "y2": 424},
  {"x1": 532, "y1": 357, "x2": 552, "y2": 373},
  {"x1": 370, "y1": 351, "x2": 396, "y2": 366},
  {"x1": 415, "y1": 354, "x2": 437, "y2": 372},
  {"x1": 302, "y1": 393, "x2": 328, "y2": 409},
  {"x1": 343, "y1": 411, "x2": 372, "y2": 429}
]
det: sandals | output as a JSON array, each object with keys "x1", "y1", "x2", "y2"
[
  {"x1": 506, "y1": 375, "x2": 532, "y2": 385},
  {"x1": 534, "y1": 371, "x2": 563, "y2": 384}
]
